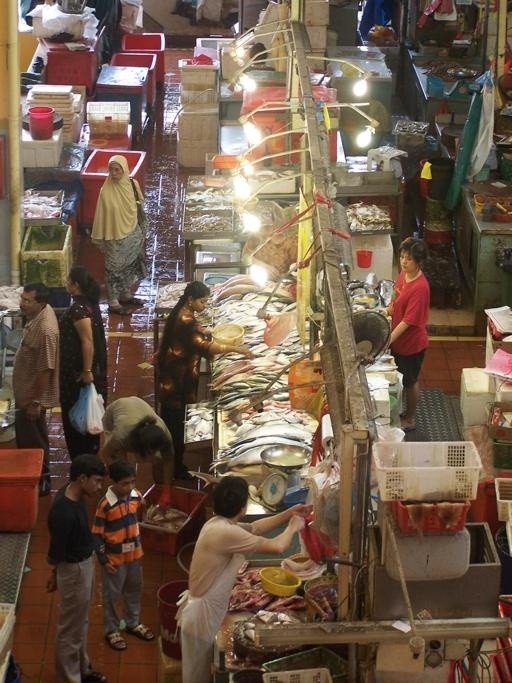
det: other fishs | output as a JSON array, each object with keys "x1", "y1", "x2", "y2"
[
  {"x1": 341, "y1": 271, "x2": 400, "y2": 373},
  {"x1": 182, "y1": 273, "x2": 315, "y2": 488},
  {"x1": 143, "y1": 504, "x2": 188, "y2": 531}
]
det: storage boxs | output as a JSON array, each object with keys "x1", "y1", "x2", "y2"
[
  {"x1": 392, "y1": 120, "x2": 430, "y2": 147},
  {"x1": 136, "y1": 482, "x2": 208, "y2": 556},
  {"x1": 460, "y1": 317, "x2": 512, "y2": 426},
  {"x1": 0, "y1": 448, "x2": 44, "y2": 533}
]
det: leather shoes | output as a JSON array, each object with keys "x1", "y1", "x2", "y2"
[{"x1": 84, "y1": 671, "x2": 107, "y2": 683}]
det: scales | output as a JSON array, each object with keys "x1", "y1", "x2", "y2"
[
  {"x1": 259, "y1": 443, "x2": 312, "y2": 512},
  {"x1": 445, "y1": 67, "x2": 478, "y2": 102}
]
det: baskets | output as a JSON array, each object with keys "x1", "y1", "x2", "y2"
[
  {"x1": 263, "y1": 669, "x2": 332, "y2": 683},
  {"x1": 495, "y1": 478, "x2": 512, "y2": 521},
  {"x1": 373, "y1": 441, "x2": 482, "y2": 502}
]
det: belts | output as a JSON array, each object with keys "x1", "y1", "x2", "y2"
[{"x1": 64, "y1": 550, "x2": 92, "y2": 563}]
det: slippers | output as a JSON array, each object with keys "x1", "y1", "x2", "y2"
[
  {"x1": 126, "y1": 623, "x2": 154, "y2": 640},
  {"x1": 107, "y1": 632, "x2": 127, "y2": 650},
  {"x1": 108, "y1": 298, "x2": 144, "y2": 315}
]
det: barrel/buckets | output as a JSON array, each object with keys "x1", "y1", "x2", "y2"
[
  {"x1": 494, "y1": 524, "x2": 512, "y2": 595},
  {"x1": 27, "y1": 106, "x2": 54, "y2": 141},
  {"x1": 419, "y1": 158, "x2": 428, "y2": 198},
  {"x1": 356, "y1": 249, "x2": 373, "y2": 268},
  {"x1": 427, "y1": 156, "x2": 455, "y2": 200},
  {"x1": 156, "y1": 579, "x2": 190, "y2": 660},
  {"x1": 175, "y1": 542, "x2": 197, "y2": 583}
]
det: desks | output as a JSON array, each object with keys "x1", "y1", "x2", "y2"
[
  {"x1": 404, "y1": 45, "x2": 512, "y2": 312},
  {"x1": 326, "y1": 44, "x2": 393, "y2": 127}
]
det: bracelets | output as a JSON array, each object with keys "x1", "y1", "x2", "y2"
[
  {"x1": 220, "y1": 344, "x2": 226, "y2": 353},
  {"x1": 81, "y1": 369, "x2": 94, "y2": 372},
  {"x1": 379, "y1": 308, "x2": 388, "y2": 315}
]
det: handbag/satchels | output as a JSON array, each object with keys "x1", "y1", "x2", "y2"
[{"x1": 138, "y1": 202, "x2": 144, "y2": 225}]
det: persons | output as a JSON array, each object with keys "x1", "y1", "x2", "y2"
[
  {"x1": 154, "y1": 279, "x2": 257, "y2": 481},
  {"x1": 97, "y1": 395, "x2": 178, "y2": 508},
  {"x1": 173, "y1": 476, "x2": 315, "y2": 683},
  {"x1": 378, "y1": 236, "x2": 432, "y2": 433},
  {"x1": 58, "y1": 265, "x2": 109, "y2": 464},
  {"x1": 10, "y1": 281, "x2": 62, "y2": 500},
  {"x1": 90, "y1": 458, "x2": 156, "y2": 652},
  {"x1": 89, "y1": 154, "x2": 150, "y2": 317},
  {"x1": 43, "y1": 451, "x2": 109, "y2": 683},
  {"x1": 227, "y1": 42, "x2": 276, "y2": 97}
]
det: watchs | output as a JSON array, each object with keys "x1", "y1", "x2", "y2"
[{"x1": 31, "y1": 399, "x2": 40, "y2": 407}]
